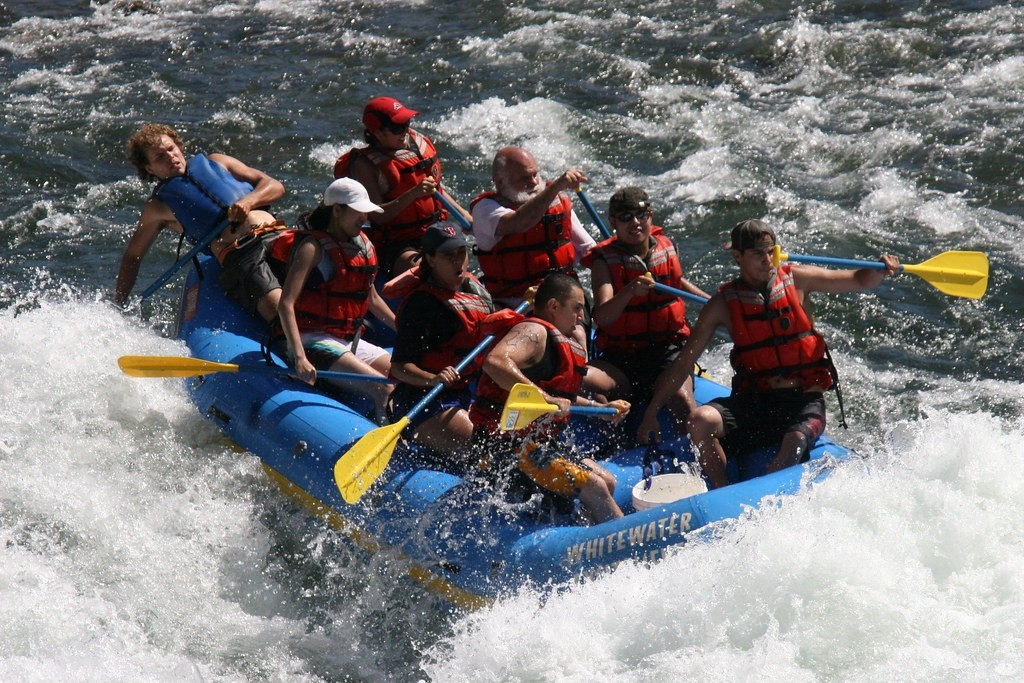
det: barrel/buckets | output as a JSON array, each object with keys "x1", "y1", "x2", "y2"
[{"x1": 631, "y1": 473, "x2": 709, "y2": 513}]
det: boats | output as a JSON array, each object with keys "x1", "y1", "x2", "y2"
[{"x1": 176, "y1": 230, "x2": 864, "y2": 623}]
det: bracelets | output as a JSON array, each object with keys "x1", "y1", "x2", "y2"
[{"x1": 587, "y1": 400, "x2": 595, "y2": 406}]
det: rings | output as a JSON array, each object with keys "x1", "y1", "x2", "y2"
[{"x1": 304, "y1": 374, "x2": 310, "y2": 380}]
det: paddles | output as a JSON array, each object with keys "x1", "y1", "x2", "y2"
[
  {"x1": 773, "y1": 244, "x2": 989, "y2": 300},
  {"x1": 642, "y1": 272, "x2": 710, "y2": 304},
  {"x1": 117, "y1": 355, "x2": 398, "y2": 385},
  {"x1": 124, "y1": 206, "x2": 237, "y2": 317},
  {"x1": 334, "y1": 297, "x2": 536, "y2": 506},
  {"x1": 427, "y1": 177, "x2": 475, "y2": 237},
  {"x1": 576, "y1": 185, "x2": 611, "y2": 239},
  {"x1": 499, "y1": 383, "x2": 621, "y2": 431}
]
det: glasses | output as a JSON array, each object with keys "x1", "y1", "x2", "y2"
[{"x1": 614, "y1": 210, "x2": 651, "y2": 221}]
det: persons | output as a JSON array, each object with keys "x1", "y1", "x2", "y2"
[
  {"x1": 114, "y1": 122, "x2": 291, "y2": 325},
  {"x1": 275, "y1": 93, "x2": 900, "y2": 524}
]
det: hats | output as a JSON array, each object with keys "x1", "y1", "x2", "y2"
[
  {"x1": 323, "y1": 176, "x2": 385, "y2": 212},
  {"x1": 362, "y1": 96, "x2": 420, "y2": 132},
  {"x1": 609, "y1": 184, "x2": 653, "y2": 218},
  {"x1": 413, "y1": 221, "x2": 472, "y2": 263},
  {"x1": 730, "y1": 218, "x2": 776, "y2": 253}
]
